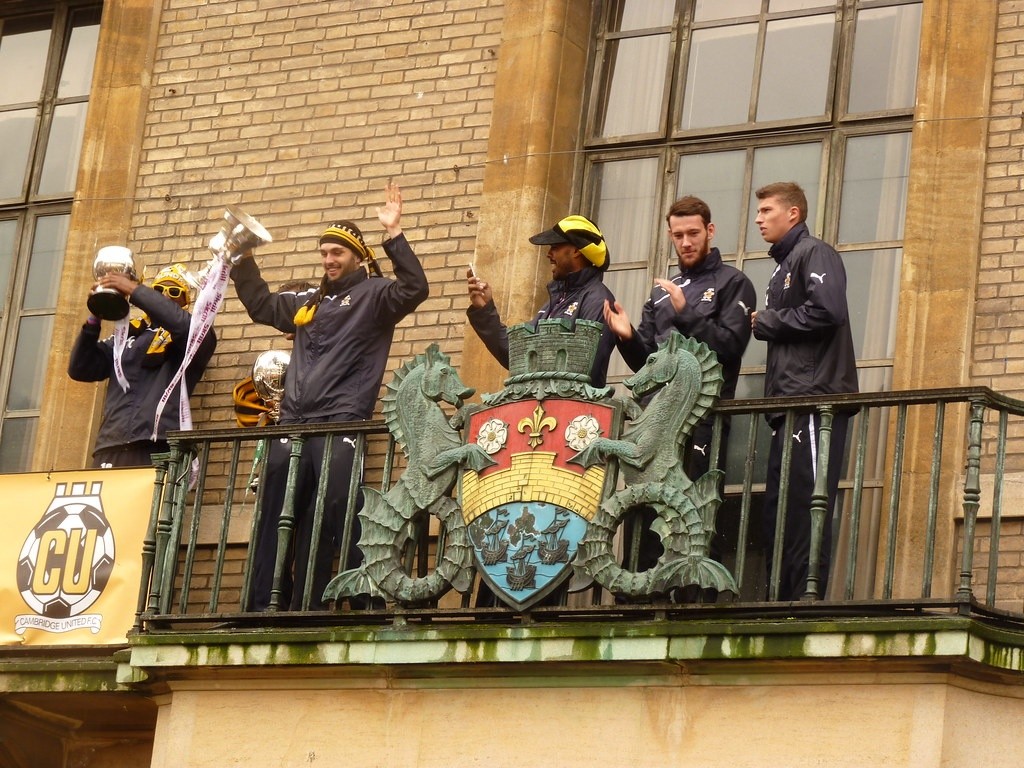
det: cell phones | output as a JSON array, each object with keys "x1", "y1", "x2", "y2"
[{"x1": 469, "y1": 263, "x2": 485, "y2": 295}]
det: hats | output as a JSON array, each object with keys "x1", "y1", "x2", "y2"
[
  {"x1": 147, "y1": 263, "x2": 192, "y2": 354},
  {"x1": 293, "y1": 220, "x2": 368, "y2": 325},
  {"x1": 529, "y1": 215, "x2": 610, "y2": 272}
]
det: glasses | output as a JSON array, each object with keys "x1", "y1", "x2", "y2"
[{"x1": 153, "y1": 283, "x2": 187, "y2": 299}]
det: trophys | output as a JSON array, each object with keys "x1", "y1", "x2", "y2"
[
  {"x1": 187, "y1": 204, "x2": 272, "y2": 292},
  {"x1": 87, "y1": 246, "x2": 146, "y2": 322},
  {"x1": 251, "y1": 349, "x2": 293, "y2": 427}
]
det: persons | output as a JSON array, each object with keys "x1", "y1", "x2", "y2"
[
  {"x1": 745, "y1": 183, "x2": 860, "y2": 601},
  {"x1": 604, "y1": 196, "x2": 756, "y2": 602},
  {"x1": 467, "y1": 215, "x2": 618, "y2": 391},
  {"x1": 208, "y1": 183, "x2": 429, "y2": 610},
  {"x1": 66, "y1": 264, "x2": 218, "y2": 612}
]
